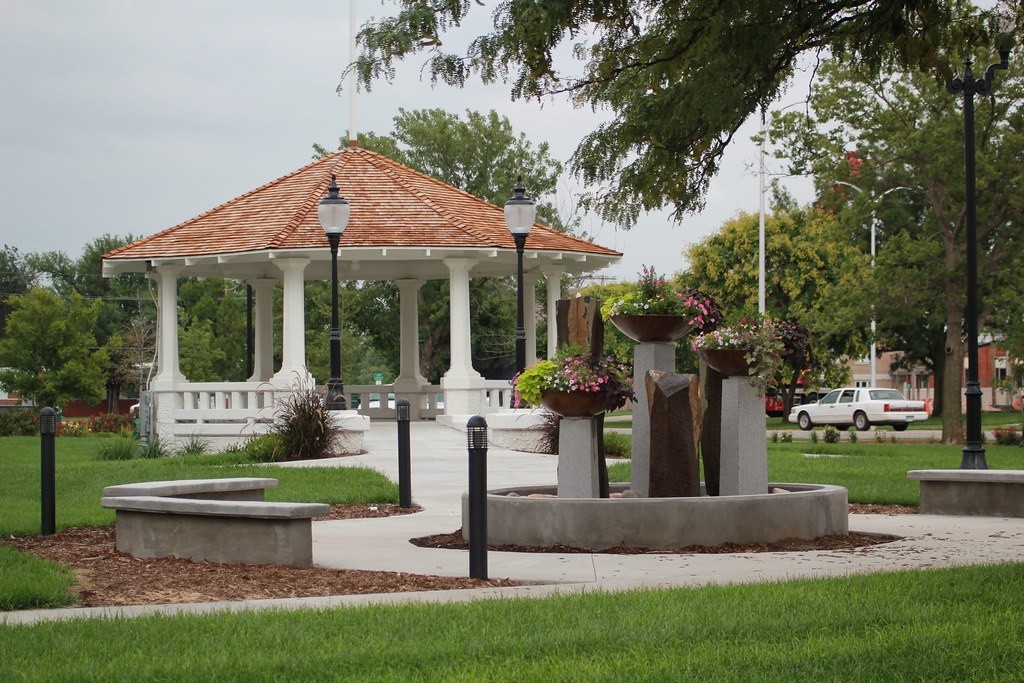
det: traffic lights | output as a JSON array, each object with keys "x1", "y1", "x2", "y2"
[{"x1": 825, "y1": 179, "x2": 917, "y2": 388}]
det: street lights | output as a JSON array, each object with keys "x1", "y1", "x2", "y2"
[
  {"x1": 503, "y1": 172, "x2": 537, "y2": 410},
  {"x1": 318, "y1": 172, "x2": 346, "y2": 412}
]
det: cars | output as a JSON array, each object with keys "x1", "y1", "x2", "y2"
[{"x1": 787, "y1": 387, "x2": 928, "y2": 431}]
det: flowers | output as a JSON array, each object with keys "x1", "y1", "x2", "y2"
[
  {"x1": 690, "y1": 311, "x2": 788, "y2": 353},
  {"x1": 599, "y1": 264, "x2": 727, "y2": 337},
  {"x1": 508, "y1": 341, "x2": 638, "y2": 413}
]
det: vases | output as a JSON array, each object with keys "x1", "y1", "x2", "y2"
[
  {"x1": 694, "y1": 348, "x2": 763, "y2": 377},
  {"x1": 540, "y1": 391, "x2": 620, "y2": 420},
  {"x1": 608, "y1": 314, "x2": 696, "y2": 344}
]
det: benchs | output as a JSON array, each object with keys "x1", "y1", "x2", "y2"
[
  {"x1": 104, "y1": 479, "x2": 278, "y2": 501},
  {"x1": 101, "y1": 495, "x2": 331, "y2": 570},
  {"x1": 910, "y1": 469, "x2": 1024, "y2": 519}
]
